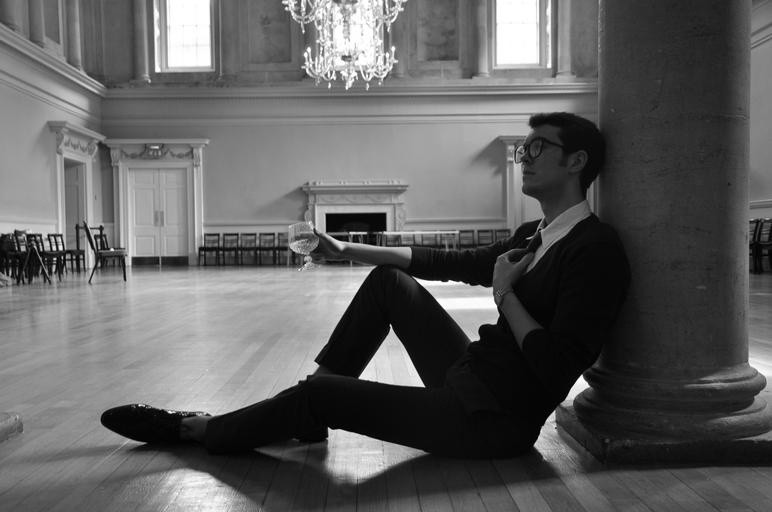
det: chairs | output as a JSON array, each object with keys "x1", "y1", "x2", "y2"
[
  {"x1": 748, "y1": 216, "x2": 772, "y2": 275},
  {"x1": 348, "y1": 227, "x2": 512, "y2": 268},
  {"x1": 0, "y1": 223, "x2": 128, "y2": 285},
  {"x1": 196, "y1": 233, "x2": 299, "y2": 268}
]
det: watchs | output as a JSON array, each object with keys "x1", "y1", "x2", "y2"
[{"x1": 494, "y1": 287, "x2": 515, "y2": 307}]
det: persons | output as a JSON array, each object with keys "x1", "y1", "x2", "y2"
[{"x1": 101, "y1": 112, "x2": 632, "y2": 460}]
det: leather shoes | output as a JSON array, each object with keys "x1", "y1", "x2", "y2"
[{"x1": 101, "y1": 403, "x2": 212, "y2": 443}]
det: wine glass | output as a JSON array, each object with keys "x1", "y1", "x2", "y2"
[{"x1": 288, "y1": 222, "x2": 321, "y2": 272}]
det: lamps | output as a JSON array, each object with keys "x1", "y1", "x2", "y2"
[{"x1": 280, "y1": 0, "x2": 404, "y2": 91}]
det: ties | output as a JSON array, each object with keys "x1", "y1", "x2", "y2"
[{"x1": 521, "y1": 228, "x2": 542, "y2": 273}]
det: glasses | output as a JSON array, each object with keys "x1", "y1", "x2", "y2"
[{"x1": 514, "y1": 137, "x2": 565, "y2": 163}]
